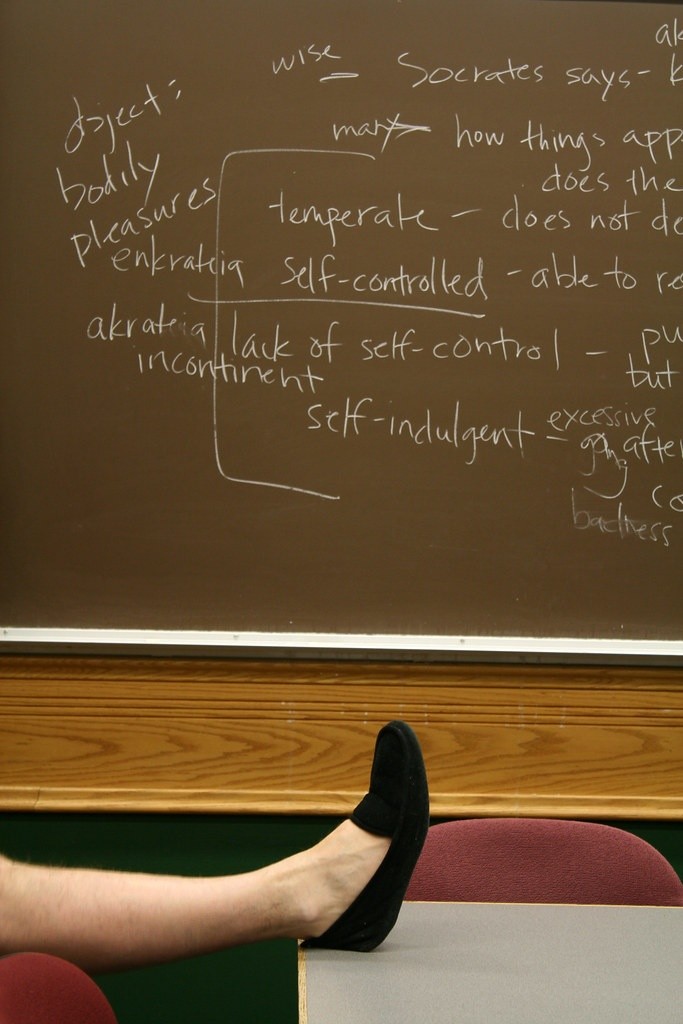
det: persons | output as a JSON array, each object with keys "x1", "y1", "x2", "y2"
[{"x1": 0, "y1": 720, "x2": 430, "y2": 964}]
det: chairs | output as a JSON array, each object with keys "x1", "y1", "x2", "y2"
[{"x1": 403, "y1": 819, "x2": 683, "y2": 906}]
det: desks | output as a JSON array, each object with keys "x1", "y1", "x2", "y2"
[{"x1": 298, "y1": 899, "x2": 683, "y2": 1024}]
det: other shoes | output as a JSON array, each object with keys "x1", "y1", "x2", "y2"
[{"x1": 298, "y1": 720, "x2": 430, "y2": 953}]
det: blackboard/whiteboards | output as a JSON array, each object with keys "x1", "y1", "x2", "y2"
[{"x1": 0, "y1": 0, "x2": 683, "y2": 670}]
